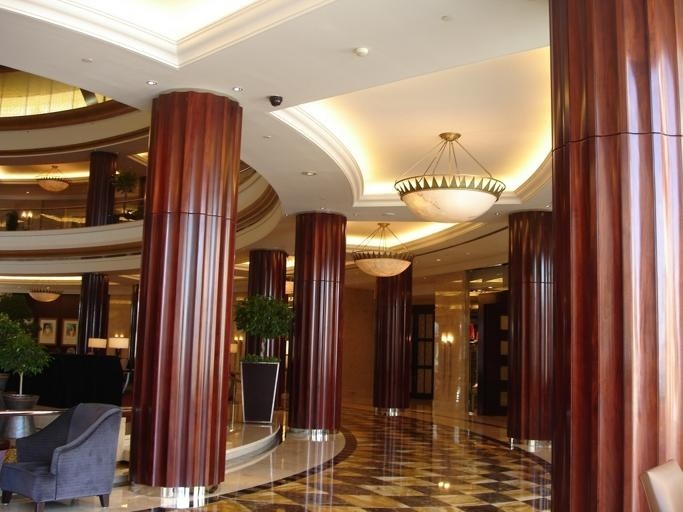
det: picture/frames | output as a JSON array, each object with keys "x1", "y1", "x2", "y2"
[
  {"x1": 36, "y1": 315, "x2": 56, "y2": 346},
  {"x1": 60, "y1": 318, "x2": 79, "y2": 345}
]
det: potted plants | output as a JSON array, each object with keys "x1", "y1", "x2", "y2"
[
  {"x1": 233, "y1": 294, "x2": 295, "y2": 425},
  {"x1": 0, "y1": 311, "x2": 56, "y2": 440}
]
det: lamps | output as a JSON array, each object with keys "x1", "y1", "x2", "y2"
[
  {"x1": 87, "y1": 337, "x2": 108, "y2": 353},
  {"x1": 389, "y1": 131, "x2": 506, "y2": 224},
  {"x1": 26, "y1": 289, "x2": 64, "y2": 302},
  {"x1": 107, "y1": 336, "x2": 128, "y2": 357},
  {"x1": 351, "y1": 222, "x2": 415, "y2": 280},
  {"x1": 34, "y1": 164, "x2": 71, "y2": 193}
]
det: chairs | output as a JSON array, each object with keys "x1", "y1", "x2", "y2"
[{"x1": 0, "y1": 401, "x2": 122, "y2": 512}]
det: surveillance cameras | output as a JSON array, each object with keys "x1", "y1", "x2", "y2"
[{"x1": 269, "y1": 96, "x2": 282, "y2": 106}]
